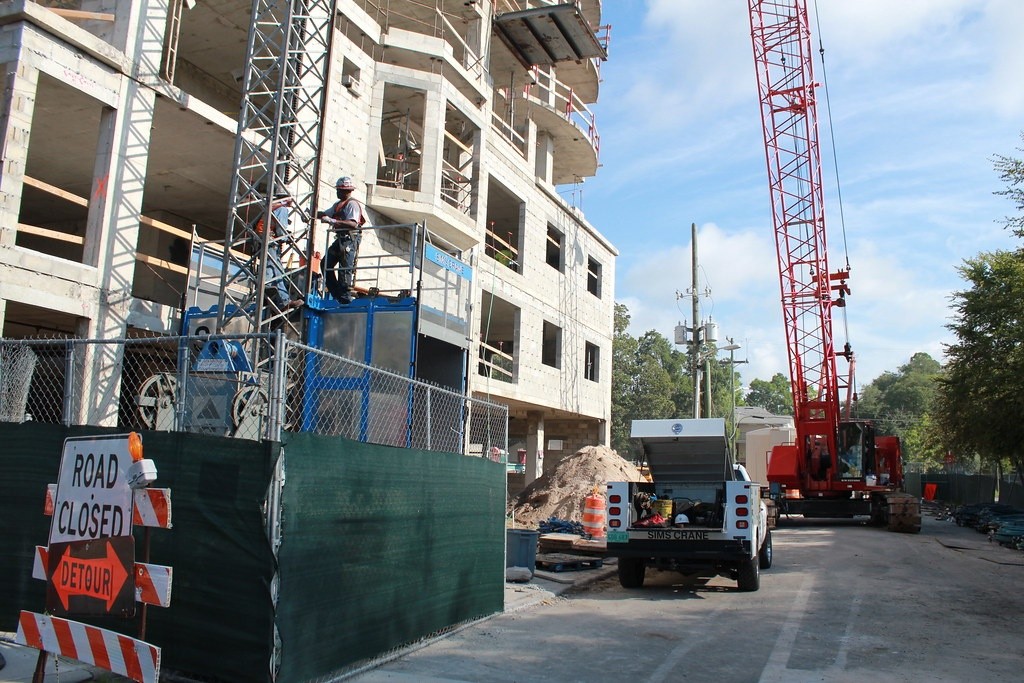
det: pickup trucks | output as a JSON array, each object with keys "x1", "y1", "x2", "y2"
[{"x1": 605, "y1": 412, "x2": 779, "y2": 591}]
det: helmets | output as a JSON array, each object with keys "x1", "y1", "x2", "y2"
[
  {"x1": 276, "y1": 184, "x2": 295, "y2": 196},
  {"x1": 335, "y1": 177, "x2": 355, "y2": 190}
]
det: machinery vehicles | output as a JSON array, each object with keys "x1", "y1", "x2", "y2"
[{"x1": 746, "y1": 0, "x2": 924, "y2": 531}]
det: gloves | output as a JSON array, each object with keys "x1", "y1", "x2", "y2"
[
  {"x1": 301, "y1": 211, "x2": 312, "y2": 222},
  {"x1": 321, "y1": 216, "x2": 337, "y2": 224}
]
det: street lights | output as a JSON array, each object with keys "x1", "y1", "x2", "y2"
[{"x1": 693, "y1": 343, "x2": 741, "y2": 418}]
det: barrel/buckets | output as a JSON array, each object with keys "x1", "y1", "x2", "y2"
[
  {"x1": 655, "y1": 499, "x2": 672, "y2": 524},
  {"x1": 508, "y1": 528, "x2": 539, "y2": 580}
]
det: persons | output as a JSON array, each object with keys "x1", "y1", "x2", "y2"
[
  {"x1": 495, "y1": 244, "x2": 515, "y2": 268},
  {"x1": 253, "y1": 185, "x2": 303, "y2": 311},
  {"x1": 848, "y1": 438, "x2": 869, "y2": 471},
  {"x1": 300, "y1": 176, "x2": 366, "y2": 305}
]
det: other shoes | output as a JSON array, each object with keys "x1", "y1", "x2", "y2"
[
  {"x1": 339, "y1": 298, "x2": 352, "y2": 303},
  {"x1": 283, "y1": 300, "x2": 305, "y2": 311}
]
